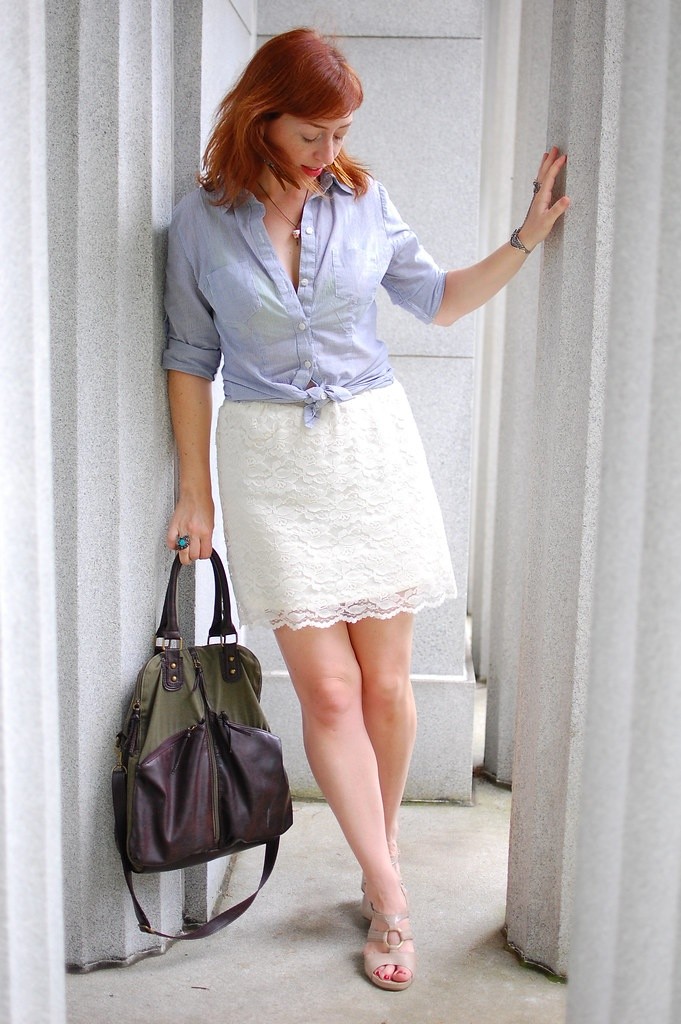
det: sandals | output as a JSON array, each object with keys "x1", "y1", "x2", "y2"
[
  {"x1": 364, "y1": 885, "x2": 413, "y2": 990},
  {"x1": 360, "y1": 878, "x2": 410, "y2": 920}
]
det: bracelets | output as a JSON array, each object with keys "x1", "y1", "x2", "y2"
[{"x1": 510, "y1": 229, "x2": 530, "y2": 254}]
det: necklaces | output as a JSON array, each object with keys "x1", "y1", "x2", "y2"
[{"x1": 255, "y1": 180, "x2": 310, "y2": 245}]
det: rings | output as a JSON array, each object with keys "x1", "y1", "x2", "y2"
[
  {"x1": 532, "y1": 181, "x2": 540, "y2": 193},
  {"x1": 177, "y1": 535, "x2": 190, "y2": 550}
]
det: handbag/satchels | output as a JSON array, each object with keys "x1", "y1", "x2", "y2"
[
  {"x1": 122, "y1": 548, "x2": 270, "y2": 872},
  {"x1": 127, "y1": 713, "x2": 295, "y2": 865}
]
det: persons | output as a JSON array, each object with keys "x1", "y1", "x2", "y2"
[{"x1": 161, "y1": 30, "x2": 571, "y2": 992}]
